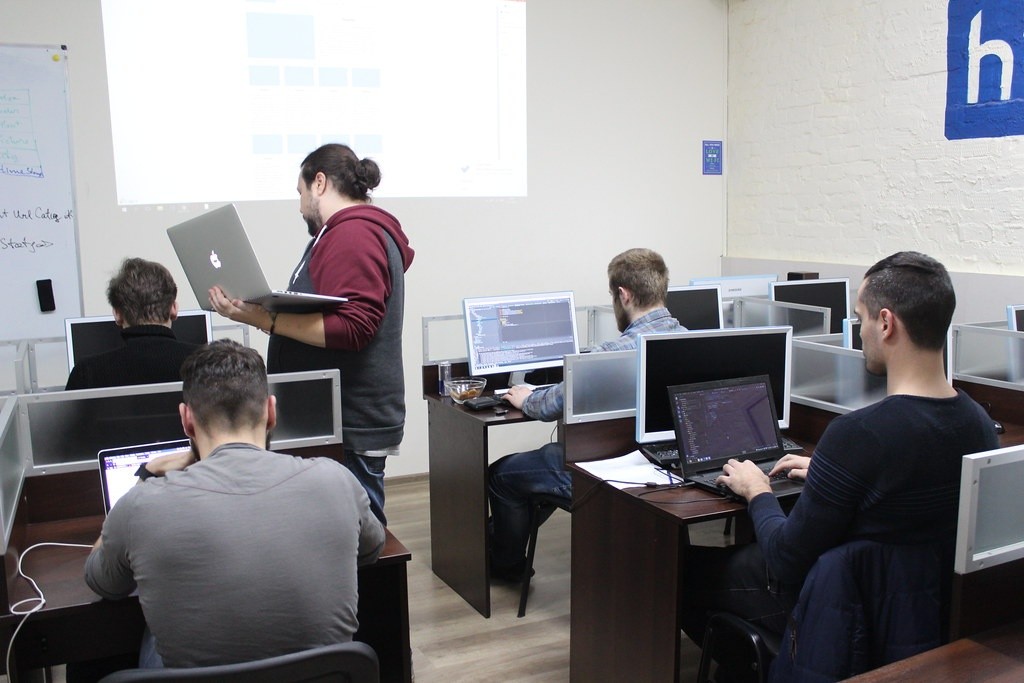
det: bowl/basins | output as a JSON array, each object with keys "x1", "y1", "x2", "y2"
[{"x1": 443, "y1": 376, "x2": 487, "y2": 404}]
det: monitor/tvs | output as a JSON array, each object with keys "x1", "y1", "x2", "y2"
[
  {"x1": 842, "y1": 318, "x2": 863, "y2": 351},
  {"x1": 664, "y1": 284, "x2": 724, "y2": 330},
  {"x1": 635, "y1": 324, "x2": 793, "y2": 442},
  {"x1": 1007, "y1": 304, "x2": 1024, "y2": 383},
  {"x1": 769, "y1": 278, "x2": 851, "y2": 335},
  {"x1": 463, "y1": 291, "x2": 580, "y2": 394},
  {"x1": 65, "y1": 309, "x2": 213, "y2": 372}
]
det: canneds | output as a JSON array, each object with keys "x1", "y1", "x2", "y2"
[{"x1": 439, "y1": 361, "x2": 452, "y2": 396}]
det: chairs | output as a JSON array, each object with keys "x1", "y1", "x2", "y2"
[
  {"x1": 515, "y1": 492, "x2": 572, "y2": 616},
  {"x1": 697, "y1": 533, "x2": 978, "y2": 683},
  {"x1": 94, "y1": 641, "x2": 380, "y2": 683}
]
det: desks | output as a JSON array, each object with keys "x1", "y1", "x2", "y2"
[
  {"x1": 423, "y1": 379, "x2": 564, "y2": 616},
  {"x1": 562, "y1": 352, "x2": 1024, "y2": 683},
  {"x1": 0, "y1": 461, "x2": 412, "y2": 683},
  {"x1": 834, "y1": 620, "x2": 1024, "y2": 683}
]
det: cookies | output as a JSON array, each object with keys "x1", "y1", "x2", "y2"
[{"x1": 452, "y1": 389, "x2": 477, "y2": 400}]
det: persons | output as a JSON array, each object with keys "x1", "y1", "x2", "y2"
[
  {"x1": 84, "y1": 337, "x2": 387, "y2": 673},
  {"x1": 486, "y1": 248, "x2": 690, "y2": 579},
  {"x1": 677, "y1": 251, "x2": 998, "y2": 683},
  {"x1": 65, "y1": 258, "x2": 204, "y2": 389},
  {"x1": 209, "y1": 143, "x2": 414, "y2": 531}
]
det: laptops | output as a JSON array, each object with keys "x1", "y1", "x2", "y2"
[
  {"x1": 97, "y1": 439, "x2": 196, "y2": 518},
  {"x1": 166, "y1": 203, "x2": 348, "y2": 313},
  {"x1": 665, "y1": 374, "x2": 806, "y2": 508}
]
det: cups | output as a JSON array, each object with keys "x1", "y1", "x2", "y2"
[{"x1": 438, "y1": 360, "x2": 452, "y2": 397}]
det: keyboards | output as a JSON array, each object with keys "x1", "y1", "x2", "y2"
[
  {"x1": 493, "y1": 384, "x2": 554, "y2": 407},
  {"x1": 644, "y1": 437, "x2": 804, "y2": 468}
]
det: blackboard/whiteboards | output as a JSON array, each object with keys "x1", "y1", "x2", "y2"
[{"x1": 0, "y1": 40, "x2": 85, "y2": 347}]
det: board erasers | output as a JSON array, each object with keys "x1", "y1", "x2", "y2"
[{"x1": 36, "y1": 279, "x2": 56, "y2": 312}]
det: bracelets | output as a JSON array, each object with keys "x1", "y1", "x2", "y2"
[
  {"x1": 134, "y1": 462, "x2": 157, "y2": 481},
  {"x1": 270, "y1": 312, "x2": 278, "y2": 334}
]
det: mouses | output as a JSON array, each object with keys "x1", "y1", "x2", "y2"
[{"x1": 991, "y1": 419, "x2": 1005, "y2": 434}]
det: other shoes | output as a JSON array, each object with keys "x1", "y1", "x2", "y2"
[{"x1": 490, "y1": 558, "x2": 536, "y2": 583}]
